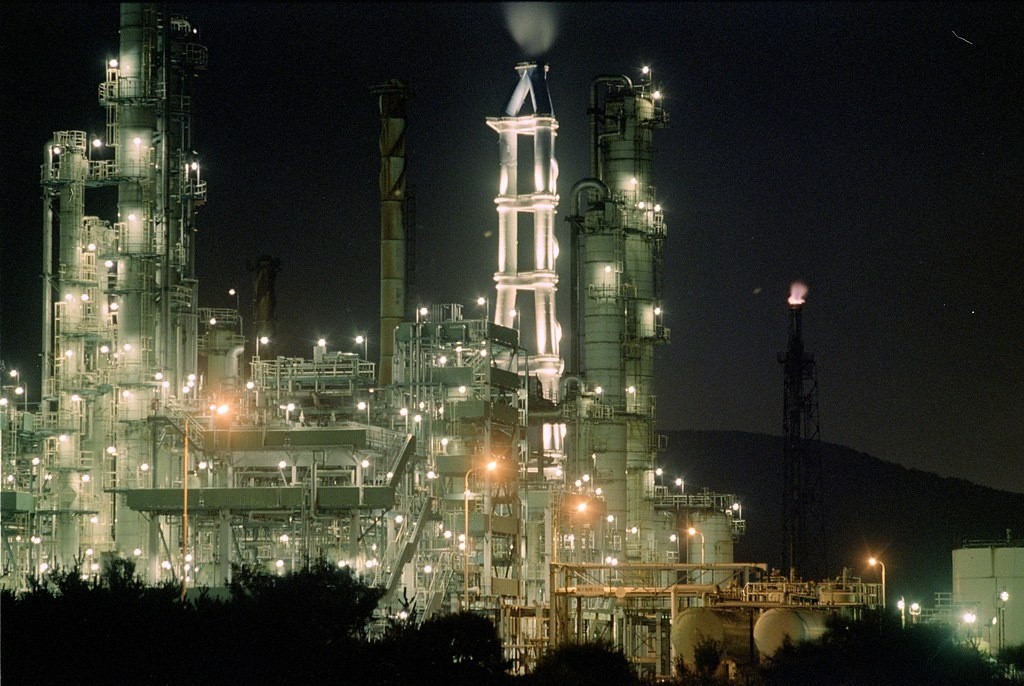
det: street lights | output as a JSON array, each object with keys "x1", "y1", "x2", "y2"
[{"x1": 1, "y1": 349, "x2": 711, "y2": 686}]
